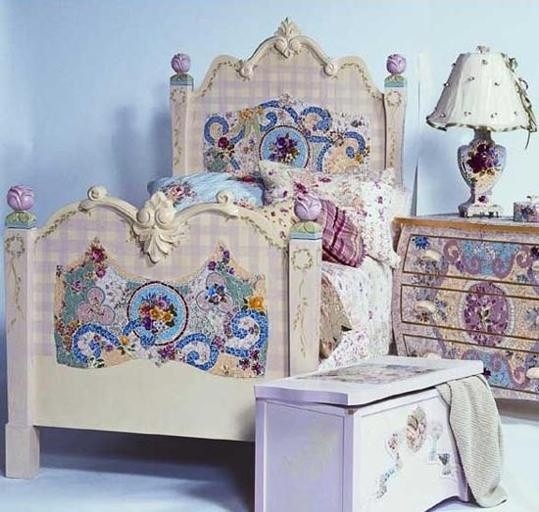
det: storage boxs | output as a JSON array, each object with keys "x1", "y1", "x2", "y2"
[{"x1": 255, "y1": 356, "x2": 492, "y2": 512}]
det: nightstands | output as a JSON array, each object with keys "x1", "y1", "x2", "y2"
[{"x1": 393, "y1": 209, "x2": 539, "y2": 403}]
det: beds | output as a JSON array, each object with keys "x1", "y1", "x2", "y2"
[{"x1": 4, "y1": 17, "x2": 403, "y2": 484}]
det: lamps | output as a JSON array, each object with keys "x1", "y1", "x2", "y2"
[{"x1": 424, "y1": 51, "x2": 536, "y2": 218}]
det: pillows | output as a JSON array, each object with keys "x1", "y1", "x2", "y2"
[{"x1": 145, "y1": 160, "x2": 395, "y2": 257}]
